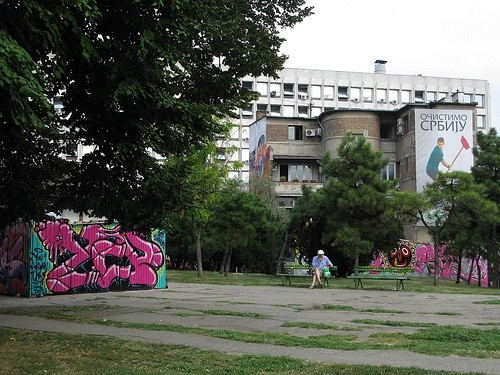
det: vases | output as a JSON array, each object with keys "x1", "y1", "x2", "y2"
[
  {"x1": 310, "y1": 179, "x2": 317, "y2": 183},
  {"x1": 302, "y1": 179, "x2": 308, "y2": 183}
]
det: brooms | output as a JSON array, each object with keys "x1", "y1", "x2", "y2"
[{"x1": 445, "y1": 135, "x2": 471, "y2": 173}]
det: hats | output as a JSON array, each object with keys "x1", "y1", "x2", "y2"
[{"x1": 317, "y1": 249, "x2": 325, "y2": 254}]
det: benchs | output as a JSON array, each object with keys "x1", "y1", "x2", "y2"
[
  {"x1": 347, "y1": 266, "x2": 414, "y2": 291},
  {"x1": 275, "y1": 264, "x2": 338, "y2": 288}
]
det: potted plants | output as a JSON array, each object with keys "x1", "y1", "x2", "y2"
[
  {"x1": 293, "y1": 176, "x2": 299, "y2": 182},
  {"x1": 280, "y1": 176, "x2": 286, "y2": 181}
]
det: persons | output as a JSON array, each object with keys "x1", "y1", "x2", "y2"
[
  {"x1": 308, "y1": 250, "x2": 334, "y2": 289},
  {"x1": 426, "y1": 137, "x2": 451, "y2": 182}
]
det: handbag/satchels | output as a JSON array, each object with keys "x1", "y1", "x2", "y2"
[{"x1": 323, "y1": 266, "x2": 331, "y2": 278}]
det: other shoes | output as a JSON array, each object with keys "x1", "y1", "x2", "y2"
[
  {"x1": 308, "y1": 284, "x2": 314, "y2": 289},
  {"x1": 318, "y1": 281, "x2": 323, "y2": 285}
]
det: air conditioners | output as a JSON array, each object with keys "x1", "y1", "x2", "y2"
[
  {"x1": 270, "y1": 90, "x2": 397, "y2": 103},
  {"x1": 306, "y1": 129, "x2": 316, "y2": 137},
  {"x1": 316, "y1": 127, "x2": 322, "y2": 136},
  {"x1": 396, "y1": 126, "x2": 405, "y2": 135},
  {"x1": 397, "y1": 118, "x2": 404, "y2": 127}
]
